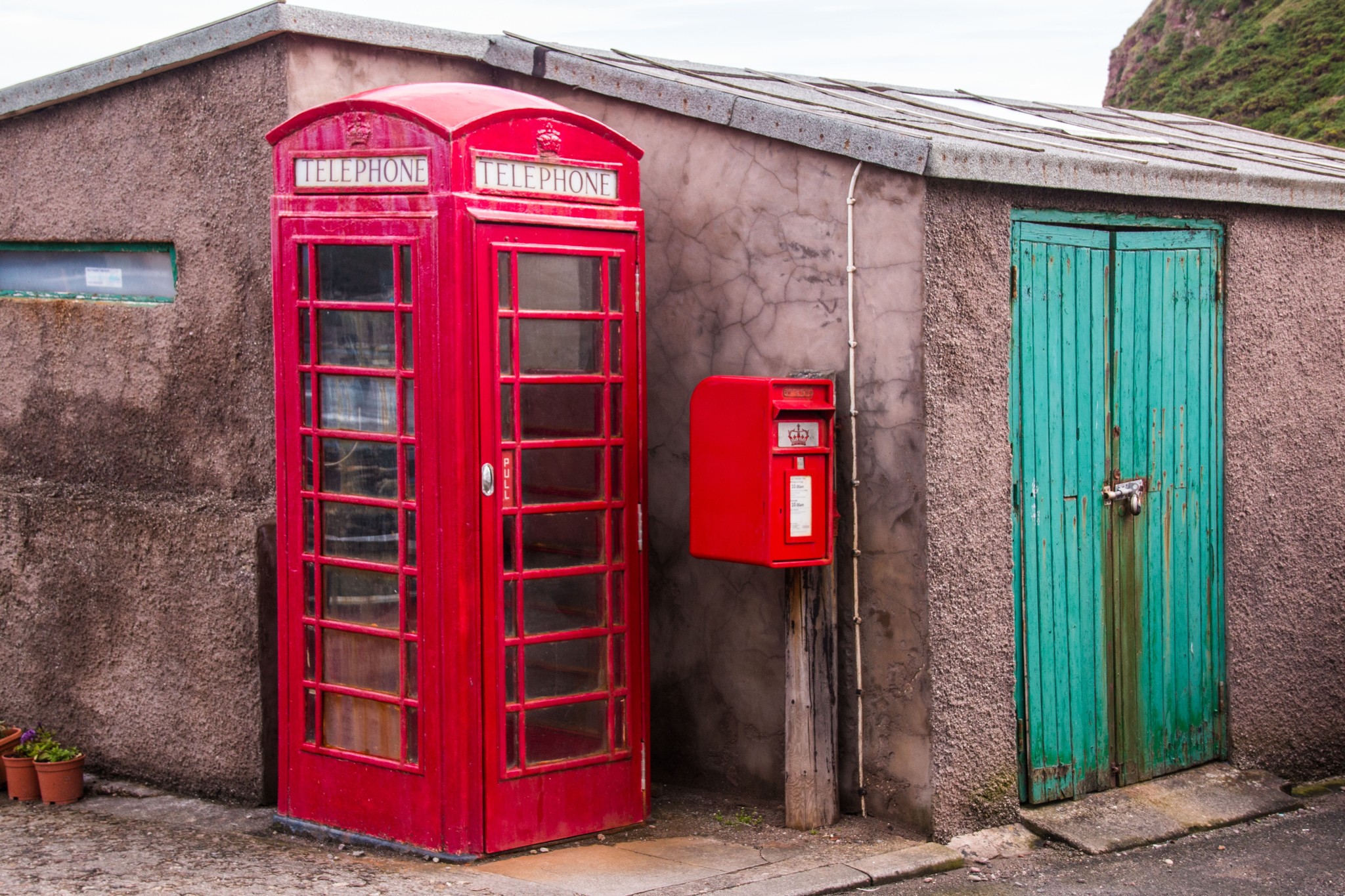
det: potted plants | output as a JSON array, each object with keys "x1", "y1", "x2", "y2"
[
  {"x1": 0, "y1": 720, "x2": 22, "y2": 783},
  {"x1": 28, "y1": 738, "x2": 86, "y2": 805}
]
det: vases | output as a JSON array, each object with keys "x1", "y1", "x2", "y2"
[{"x1": 2, "y1": 752, "x2": 40, "y2": 800}]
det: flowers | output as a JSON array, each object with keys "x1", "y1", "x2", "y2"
[{"x1": 12, "y1": 728, "x2": 57, "y2": 758}]
